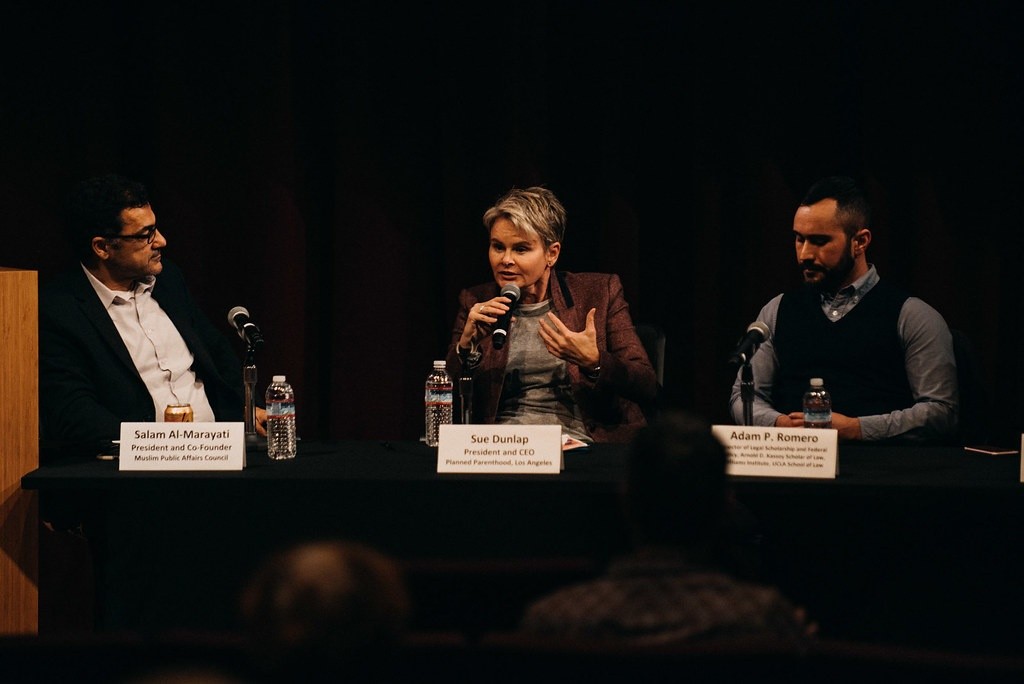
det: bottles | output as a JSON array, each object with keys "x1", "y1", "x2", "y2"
[
  {"x1": 803, "y1": 378, "x2": 831, "y2": 429},
  {"x1": 425, "y1": 361, "x2": 453, "y2": 447},
  {"x1": 266, "y1": 376, "x2": 297, "y2": 460}
]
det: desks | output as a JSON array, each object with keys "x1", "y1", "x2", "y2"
[{"x1": 20, "y1": 439, "x2": 1024, "y2": 658}]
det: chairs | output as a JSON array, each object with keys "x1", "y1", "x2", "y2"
[{"x1": 635, "y1": 322, "x2": 668, "y2": 385}]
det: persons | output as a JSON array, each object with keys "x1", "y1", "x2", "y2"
[
  {"x1": 729, "y1": 175, "x2": 959, "y2": 443},
  {"x1": 39, "y1": 174, "x2": 271, "y2": 456},
  {"x1": 445, "y1": 186, "x2": 656, "y2": 444}
]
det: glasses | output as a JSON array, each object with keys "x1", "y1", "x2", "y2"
[{"x1": 97, "y1": 223, "x2": 162, "y2": 242}]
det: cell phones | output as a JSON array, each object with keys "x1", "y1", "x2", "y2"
[
  {"x1": 96, "y1": 440, "x2": 120, "y2": 461},
  {"x1": 964, "y1": 444, "x2": 1019, "y2": 455}
]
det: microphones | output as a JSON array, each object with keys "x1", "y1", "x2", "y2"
[
  {"x1": 491, "y1": 283, "x2": 521, "y2": 350},
  {"x1": 728, "y1": 321, "x2": 770, "y2": 370},
  {"x1": 227, "y1": 306, "x2": 264, "y2": 348}
]
